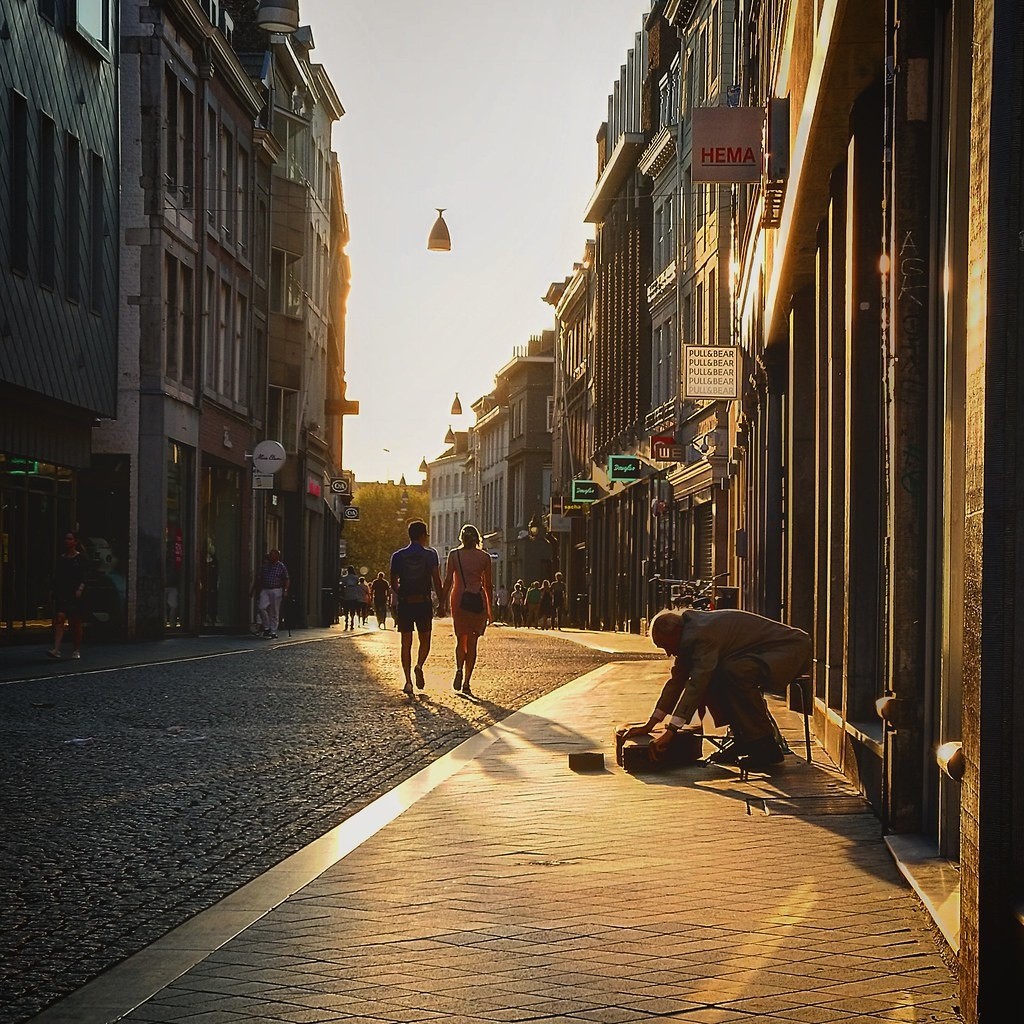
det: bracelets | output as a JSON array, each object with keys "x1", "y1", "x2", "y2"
[
  {"x1": 77, "y1": 588, "x2": 83, "y2": 592},
  {"x1": 80, "y1": 585, "x2": 85, "y2": 589},
  {"x1": 665, "y1": 724, "x2": 678, "y2": 736}
]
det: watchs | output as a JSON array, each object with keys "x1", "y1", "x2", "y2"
[{"x1": 283, "y1": 588, "x2": 288, "y2": 591}]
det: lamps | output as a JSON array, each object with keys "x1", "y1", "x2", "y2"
[
  {"x1": 451, "y1": 393, "x2": 462, "y2": 415},
  {"x1": 700, "y1": 432, "x2": 718, "y2": 453},
  {"x1": 396, "y1": 488, "x2": 409, "y2": 521},
  {"x1": 445, "y1": 425, "x2": 455, "y2": 443},
  {"x1": 256, "y1": 0, "x2": 300, "y2": 33},
  {"x1": 398, "y1": 472, "x2": 406, "y2": 487},
  {"x1": 528, "y1": 513, "x2": 540, "y2": 536},
  {"x1": 427, "y1": 208, "x2": 452, "y2": 252}
]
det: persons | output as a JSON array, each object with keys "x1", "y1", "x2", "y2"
[
  {"x1": 357, "y1": 577, "x2": 371, "y2": 628},
  {"x1": 389, "y1": 522, "x2": 443, "y2": 695},
  {"x1": 388, "y1": 578, "x2": 400, "y2": 631},
  {"x1": 46, "y1": 533, "x2": 88, "y2": 659},
  {"x1": 370, "y1": 572, "x2": 389, "y2": 629},
  {"x1": 340, "y1": 565, "x2": 358, "y2": 631},
  {"x1": 250, "y1": 549, "x2": 290, "y2": 638},
  {"x1": 496, "y1": 585, "x2": 510, "y2": 623},
  {"x1": 438, "y1": 524, "x2": 493, "y2": 693},
  {"x1": 621, "y1": 610, "x2": 813, "y2": 768},
  {"x1": 508, "y1": 572, "x2": 566, "y2": 631}
]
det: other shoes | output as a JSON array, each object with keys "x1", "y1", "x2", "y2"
[
  {"x1": 462, "y1": 685, "x2": 471, "y2": 694},
  {"x1": 48, "y1": 647, "x2": 61, "y2": 658},
  {"x1": 710, "y1": 744, "x2": 743, "y2": 764},
  {"x1": 403, "y1": 682, "x2": 413, "y2": 693},
  {"x1": 414, "y1": 664, "x2": 424, "y2": 690},
  {"x1": 71, "y1": 648, "x2": 80, "y2": 659},
  {"x1": 737, "y1": 741, "x2": 784, "y2": 768},
  {"x1": 262, "y1": 628, "x2": 278, "y2": 640},
  {"x1": 453, "y1": 670, "x2": 462, "y2": 690}
]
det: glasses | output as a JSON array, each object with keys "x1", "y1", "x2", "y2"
[{"x1": 426, "y1": 533, "x2": 429, "y2": 536}]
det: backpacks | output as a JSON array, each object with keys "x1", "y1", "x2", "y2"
[{"x1": 399, "y1": 551, "x2": 426, "y2": 595}]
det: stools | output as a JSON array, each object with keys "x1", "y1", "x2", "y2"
[{"x1": 759, "y1": 675, "x2": 813, "y2": 764}]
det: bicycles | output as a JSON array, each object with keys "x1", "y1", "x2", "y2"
[{"x1": 673, "y1": 572, "x2": 730, "y2": 611}]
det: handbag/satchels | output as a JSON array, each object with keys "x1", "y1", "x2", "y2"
[{"x1": 459, "y1": 591, "x2": 483, "y2": 613}]
